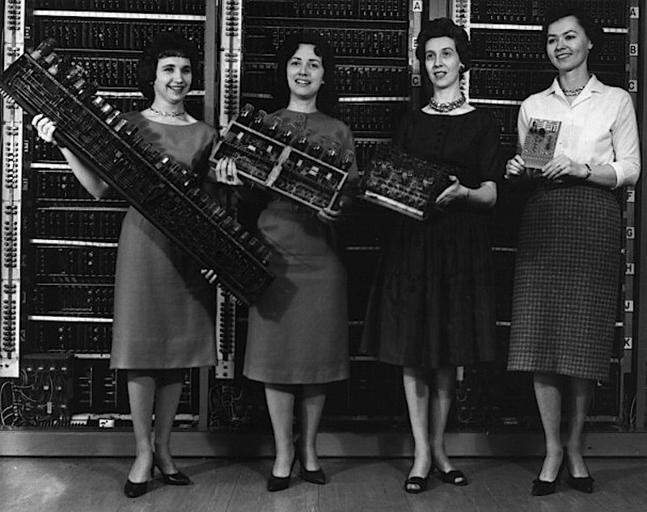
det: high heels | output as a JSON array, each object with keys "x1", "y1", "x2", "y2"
[
  {"x1": 565, "y1": 447, "x2": 594, "y2": 494},
  {"x1": 125, "y1": 454, "x2": 154, "y2": 498},
  {"x1": 530, "y1": 450, "x2": 563, "y2": 496},
  {"x1": 298, "y1": 440, "x2": 326, "y2": 485},
  {"x1": 268, "y1": 445, "x2": 297, "y2": 491},
  {"x1": 154, "y1": 454, "x2": 191, "y2": 486}
]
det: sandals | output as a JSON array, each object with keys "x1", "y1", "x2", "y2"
[
  {"x1": 432, "y1": 451, "x2": 469, "y2": 487},
  {"x1": 404, "y1": 455, "x2": 434, "y2": 493}
]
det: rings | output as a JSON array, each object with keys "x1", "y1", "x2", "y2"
[{"x1": 224, "y1": 172, "x2": 235, "y2": 183}]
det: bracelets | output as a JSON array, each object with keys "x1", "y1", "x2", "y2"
[
  {"x1": 463, "y1": 187, "x2": 473, "y2": 202},
  {"x1": 584, "y1": 161, "x2": 592, "y2": 182}
]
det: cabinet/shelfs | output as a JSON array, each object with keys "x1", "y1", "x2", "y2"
[
  {"x1": 212, "y1": 0, "x2": 439, "y2": 459},
  {"x1": 0, "y1": 0, "x2": 210, "y2": 457},
  {"x1": 440, "y1": 0, "x2": 638, "y2": 458}
]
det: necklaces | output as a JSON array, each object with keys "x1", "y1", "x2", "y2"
[
  {"x1": 430, "y1": 92, "x2": 466, "y2": 113},
  {"x1": 563, "y1": 86, "x2": 583, "y2": 99},
  {"x1": 149, "y1": 105, "x2": 185, "y2": 118}
]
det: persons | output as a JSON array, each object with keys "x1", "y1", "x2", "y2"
[
  {"x1": 30, "y1": 34, "x2": 222, "y2": 499},
  {"x1": 361, "y1": 15, "x2": 502, "y2": 494},
  {"x1": 503, "y1": 10, "x2": 642, "y2": 498},
  {"x1": 212, "y1": 29, "x2": 362, "y2": 493}
]
